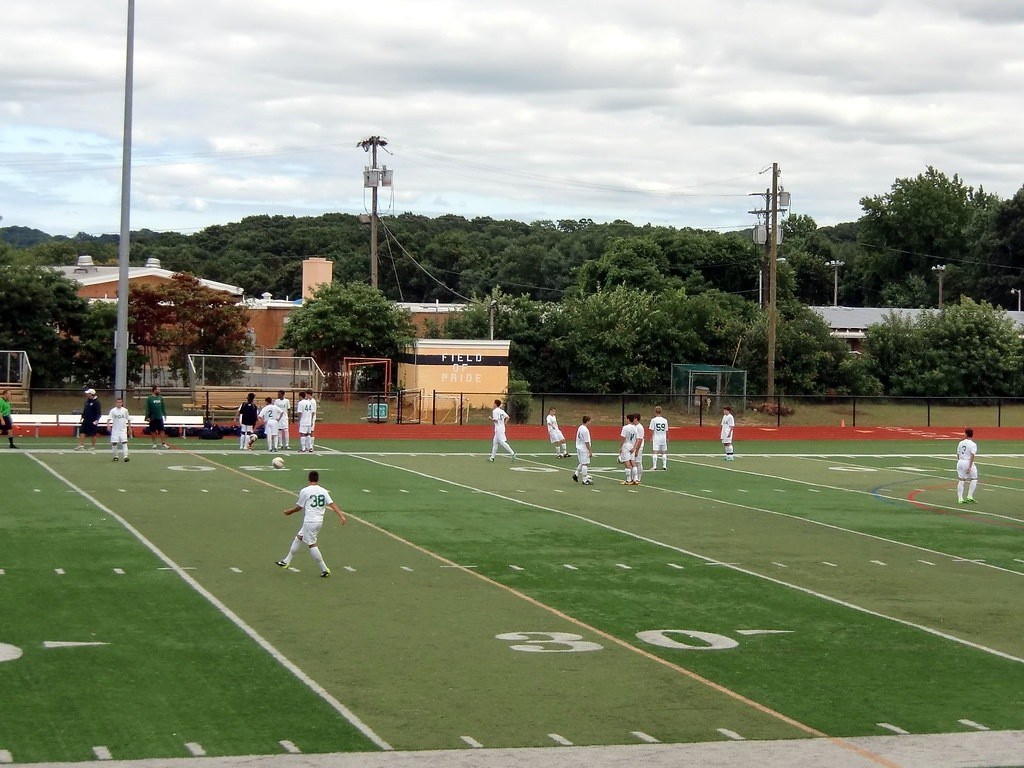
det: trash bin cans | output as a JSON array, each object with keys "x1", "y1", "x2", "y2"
[{"x1": 367, "y1": 395, "x2": 388, "y2": 423}]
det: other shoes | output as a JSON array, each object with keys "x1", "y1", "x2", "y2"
[
  {"x1": 510, "y1": 453, "x2": 517, "y2": 463},
  {"x1": 724, "y1": 455, "x2": 734, "y2": 461},
  {"x1": 620, "y1": 480, "x2": 639, "y2": 485},
  {"x1": 572, "y1": 474, "x2": 579, "y2": 483},
  {"x1": 239, "y1": 446, "x2": 248, "y2": 450},
  {"x1": 486, "y1": 458, "x2": 494, "y2": 462},
  {"x1": 124, "y1": 458, "x2": 130, "y2": 462},
  {"x1": 320, "y1": 568, "x2": 331, "y2": 577},
  {"x1": 86, "y1": 446, "x2": 95, "y2": 451},
  {"x1": 298, "y1": 449, "x2": 306, "y2": 453},
  {"x1": 112, "y1": 458, "x2": 118, "y2": 462},
  {"x1": 663, "y1": 468, "x2": 666, "y2": 470},
  {"x1": 269, "y1": 448, "x2": 277, "y2": 452},
  {"x1": 557, "y1": 452, "x2": 572, "y2": 459},
  {"x1": 581, "y1": 478, "x2": 593, "y2": 485},
  {"x1": 272, "y1": 559, "x2": 290, "y2": 569},
  {"x1": 308, "y1": 448, "x2": 313, "y2": 453},
  {"x1": 152, "y1": 443, "x2": 157, "y2": 449},
  {"x1": 9, "y1": 445, "x2": 19, "y2": 449},
  {"x1": 649, "y1": 466, "x2": 656, "y2": 470},
  {"x1": 958, "y1": 497, "x2": 978, "y2": 505},
  {"x1": 161, "y1": 443, "x2": 170, "y2": 449},
  {"x1": 74, "y1": 446, "x2": 84, "y2": 451},
  {"x1": 277, "y1": 445, "x2": 291, "y2": 449}
]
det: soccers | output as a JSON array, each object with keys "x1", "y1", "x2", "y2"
[
  {"x1": 617, "y1": 456, "x2": 625, "y2": 464},
  {"x1": 272, "y1": 457, "x2": 285, "y2": 470},
  {"x1": 587, "y1": 475, "x2": 594, "y2": 482},
  {"x1": 250, "y1": 434, "x2": 258, "y2": 441}
]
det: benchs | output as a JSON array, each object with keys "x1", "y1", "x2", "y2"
[{"x1": 0, "y1": 384, "x2": 324, "y2": 440}]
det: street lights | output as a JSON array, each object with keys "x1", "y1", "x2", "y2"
[
  {"x1": 824, "y1": 260, "x2": 845, "y2": 306},
  {"x1": 932, "y1": 264, "x2": 945, "y2": 309}
]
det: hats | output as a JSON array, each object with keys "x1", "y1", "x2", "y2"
[{"x1": 85, "y1": 388, "x2": 96, "y2": 395}]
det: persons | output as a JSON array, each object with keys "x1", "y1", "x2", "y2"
[
  {"x1": 144, "y1": 386, "x2": 170, "y2": 449},
  {"x1": 485, "y1": 400, "x2": 517, "y2": 463},
  {"x1": 296, "y1": 389, "x2": 317, "y2": 453},
  {"x1": 232, "y1": 393, "x2": 260, "y2": 450},
  {"x1": 74, "y1": 388, "x2": 102, "y2": 452},
  {"x1": 649, "y1": 406, "x2": 668, "y2": 471},
  {"x1": 956, "y1": 428, "x2": 979, "y2": 505},
  {"x1": 619, "y1": 413, "x2": 645, "y2": 484},
  {"x1": 720, "y1": 406, "x2": 735, "y2": 461},
  {"x1": 572, "y1": 416, "x2": 594, "y2": 485},
  {"x1": 0, "y1": 390, "x2": 20, "y2": 450},
  {"x1": 275, "y1": 470, "x2": 345, "y2": 578},
  {"x1": 274, "y1": 390, "x2": 292, "y2": 450},
  {"x1": 107, "y1": 398, "x2": 132, "y2": 462},
  {"x1": 546, "y1": 407, "x2": 572, "y2": 459},
  {"x1": 258, "y1": 397, "x2": 284, "y2": 451}
]
